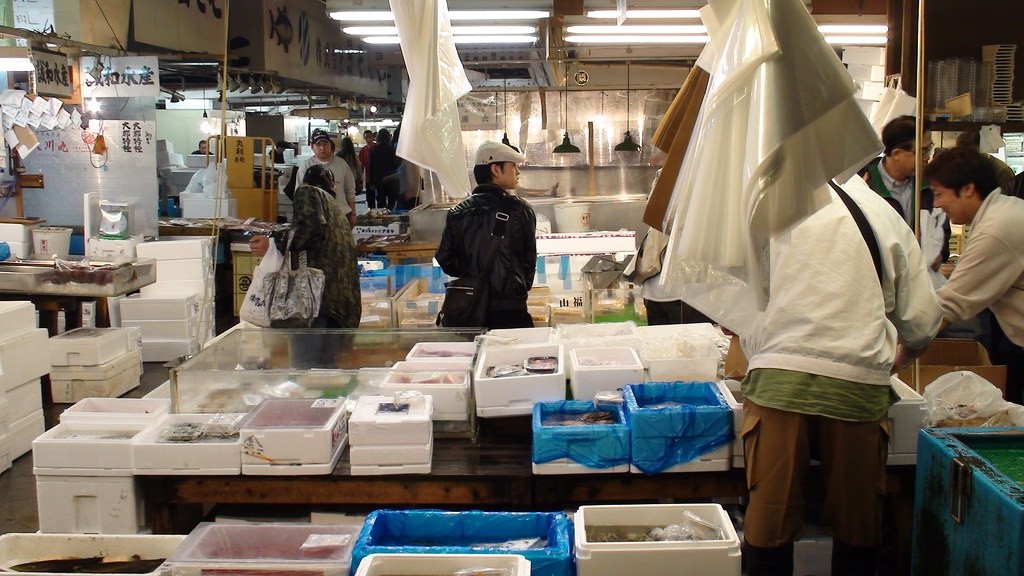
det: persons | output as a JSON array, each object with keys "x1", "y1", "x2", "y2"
[
  {"x1": 858, "y1": 115, "x2": 951, "y2": 272},
  {"x1": 335, "y1": 138, "x2": 363, "y2": 183},
  {"x1": 191, "y1": 140, "x2": 214, "y2": 155},
  {"x1": 273, "y1": 141, "x2": 289, "y2": 164},
  {"x1": 435, "y1": 141, "x2": 537, "y2": 329},
  {"x1": 294, "y1": 131, "x2": 356, "y2": 230},
  {"x1": 720, "y1": 174, "x2": 945, "y2": 576},
  {"x1": 359, "y1": 126, "x2": 423, "y2": 210},
  {"x1": 924, "y1": 147, "x2": 1024, "y2": 408},
  {"x1": 956, "y1": 131, "x2": 1015, "y2": 196},
  {"x1": 250, "y1": 165, "x2": 362, "y2": 369}
]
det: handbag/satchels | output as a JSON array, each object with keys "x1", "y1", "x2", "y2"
[
  {"x1": 622, "y1": 227, "x2": 671, "y2": 288},
  {"x1": 869, "y1": 75, "x2": 916, "y2": 142},
  {"x1": 239, "y1": 237, "x2": 285, "y2": 328},
  {"x1": 920, "y1": 208, "x2": 947, "y2": 291},
  {"x1": 355, "y1": 175, "x2": 363, "y2": 196},
  {"x1": 262, "y1": 226, "x2": 326, "y2": 329},
  {"x1": 436, "y1": 276, "x2": 489, "y2": 340}
]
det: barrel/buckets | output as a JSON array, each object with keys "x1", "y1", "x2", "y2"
[
  {"x1": 552, "y1": 202, "x2": 591, "y2": 234},
  {"x1": 31, "y1": 227, "x2": 73, "y2": 258}
]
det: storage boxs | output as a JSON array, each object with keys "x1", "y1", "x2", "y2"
[
  {"x1": 793, "y1": 528, "x2": 835, "y2": 576},
  {"x1": 168, "y1": 520, "x2": 360, "y2": 570},
  {"x1": 574, "y1": 501, "x2": 741, "y2": 575},
  {"x1": 890, "y1": 375, "x2": 929, "y2": 466},
  {"x1": 0, "y1": 237, "x2": 755, "y2": 536},
  {"x1": 157, "y1": 139, "x2": 237, "y2": 219},
  {"x1": 0, "y1": 532, "x2": 188, "y2": 576},
  {"x1": 351, "y1": 509, "x2": 572, "y2": 575},
  {"x1": 905, "y1": 338, "x2": 1007, "y2": 407},
  {"x1": 355, "y1": 552, "x2": 532, "y2": 576}
]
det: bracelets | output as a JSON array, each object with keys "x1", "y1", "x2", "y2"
[{"x1": 351, "y1": 214, "x2": 356, "y2": 216}]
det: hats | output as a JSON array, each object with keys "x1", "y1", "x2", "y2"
[
  {"x1": 312, "y1": 131, "x2": 330, "y2": 145},
  {"x1": 474, "y1": 140, "x2": 527, "y2": 166}
]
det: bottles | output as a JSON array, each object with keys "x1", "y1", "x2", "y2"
[{"x1": 51, "y1": 254, "x2": 72, "y2": 270}]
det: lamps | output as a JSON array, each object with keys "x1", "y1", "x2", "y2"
[
  {"x1": 501, "y1": 74, "x2": 519, "y2": 154},
  {"x1": 553, "y1": 61, "x2": 581, "y2": 154},
  {"x1": 614, "y1": 60, "x2": 640, "y2": 151}
]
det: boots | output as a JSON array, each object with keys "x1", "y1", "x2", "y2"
[
  {"x1": 741, "y1": 536, "x2": 794, "y2": 576},
  {"x1": 831, "y1": 537, "x2": 881, "y2": 576}
]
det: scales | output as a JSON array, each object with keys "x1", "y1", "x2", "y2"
[{"x1": 97, "y1": 201, "x2": 135, "y2": 240}]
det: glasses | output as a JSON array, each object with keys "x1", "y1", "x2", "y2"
[{"x1": 892, "y1": 142, "x2": 934, "y2": 155}]
